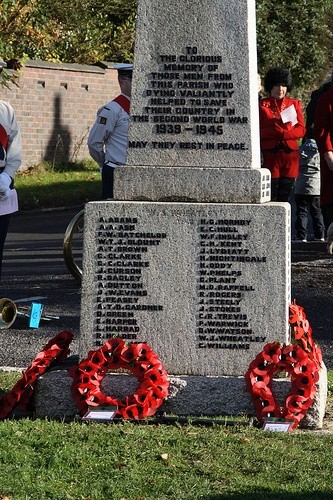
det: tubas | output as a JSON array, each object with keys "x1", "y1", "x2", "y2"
[{"x1": 0, "y1": 297, "x2": 78, "y2": 331}]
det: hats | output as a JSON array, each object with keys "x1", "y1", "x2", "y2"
[
  {"x1": 0, "y1": 61, "x2": 7, "y2": 69},
  {"x1": 114, "y1": 64, "x2": 133, "y2": 78},
  {"x1": 265, "y1": 67, "x2": 292, "y2": 92}
]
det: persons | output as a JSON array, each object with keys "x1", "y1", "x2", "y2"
[
  {"x1": 259, "y1": 68, "x2": 333, "y2": 255},
  {"x1": 0, "y1": 57, "x2": 22, "y2": 285},
  {"x1": 87, "y1": 63, "x2": 133, "y2": 200}
]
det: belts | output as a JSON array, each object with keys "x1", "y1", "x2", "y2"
[{"x1": 104, "y1": 161, "x2": 118, "y2": 168}]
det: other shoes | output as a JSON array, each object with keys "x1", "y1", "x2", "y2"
[
  {"x1": 314, "y1": 238, "x2": 324, "y2": 242},
  {"x1": 303, "y1": 240, "x2": 308, "y2": 242}
]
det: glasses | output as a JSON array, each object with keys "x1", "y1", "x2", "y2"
[{"x1": 123, "y1": 78, "x2": 132, "y2": 82}]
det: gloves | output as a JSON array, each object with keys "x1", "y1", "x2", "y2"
[
  {"x1": 0, "y1": 172, "x2": 12, "y2": 192},
  {"x1": 272, "y1": 120, "x2": 292, "y2": 139},
  {"x1": 100, "y1": 167, "x2": 102, "y2": 173}
]
